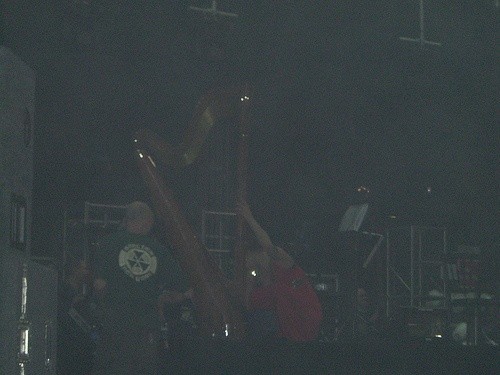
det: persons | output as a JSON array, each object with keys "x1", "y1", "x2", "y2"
[
  {"x1": 236, "y1": 197, "x2": 324, "y2": 374},
  {"x1": 85, "y1": 203, "x2": 197, "y2": 375}
]
